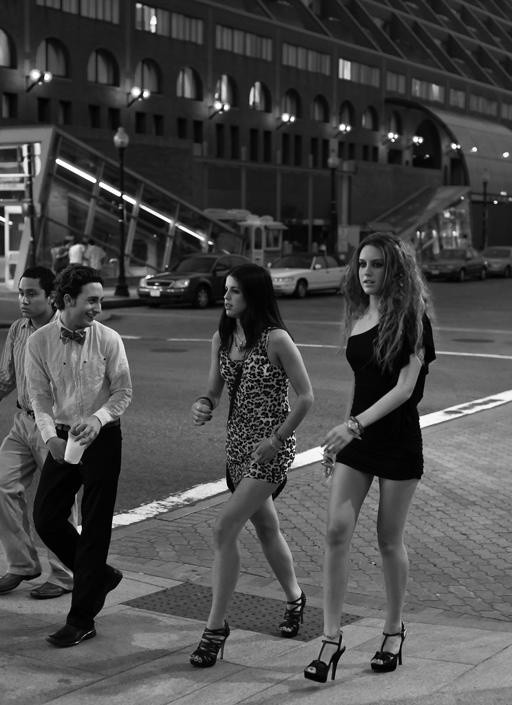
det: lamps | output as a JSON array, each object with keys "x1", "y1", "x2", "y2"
[
  {"x1": 127, "y1": 87, "x2": 150, "y2": 108},
  {"x1": 26, "y1": 70, "x2": 53, "y2": 93},
  {"x1": 333, "y1": 123, "x2": 509, "y2": 158},
  {"x1": 209, "y1": 101, "x2": 231, "y2": 120},
  {"x1": 276, "y1": 112, "x2": 296, "y2": 130}
]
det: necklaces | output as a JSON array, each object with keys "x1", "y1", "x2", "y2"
[{"x1": 236, "y1": 331, "x2": 246, "y2": 345}]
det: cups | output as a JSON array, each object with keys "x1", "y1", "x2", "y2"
[{"x1": 63, "y1": 431, "x2": 85, "y2": 465}]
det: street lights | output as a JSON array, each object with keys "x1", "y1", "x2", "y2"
[
  {"x1": 111, "y1": 124, "x2": 133, "y2": 299},
  {"x1": 325, "y1": 148, "x2": 340, "y2": 252},
  {"x1": 479, "y1": 165, "x2": 491, "y2": 248}
]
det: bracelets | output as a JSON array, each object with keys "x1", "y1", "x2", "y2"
[
  {"x1": 194, "y1": 396, "x2": 214, "y2": 411},
  {"x1": 343, "y1": 416, "x2": 365, "y2": 440}
]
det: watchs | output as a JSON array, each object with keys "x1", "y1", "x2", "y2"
[{"x1": 345, "y1": 417, "x2": 362, "y2": 436}]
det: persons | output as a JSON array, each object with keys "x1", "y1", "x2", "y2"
[
  {"x1": 0, "y1": 265, "x2": 79, "y2": 598},
  {"x1": 54, "y1": 235, "x2": 107, "y2": 277},
  {"x1": 188, "y1": 263, "x2": 315, "y2": 668},
  {"x1": 24, "y1": 262, "x2": 133, "y2": 648},
  {"x1": 302, "y1": 229, "x2": 437, "y2": 684}
]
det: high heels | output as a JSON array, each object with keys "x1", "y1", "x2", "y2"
[
  {"x1": 304, "y1": 632, "x2": 346, "y2": 683},
  {"x1": 190, "y1": 619, "x2": 230, "y2": 668},
  {"x1": 279, "y1": 591, "x2": 306, "y2": 638},
  {"x1": 371, "y1": 621, "x2": 407, "y2": 673}
]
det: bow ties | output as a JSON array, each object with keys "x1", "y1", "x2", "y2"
[{"x1": 60, "y1": 327, "x2": 87, "y2": 345}]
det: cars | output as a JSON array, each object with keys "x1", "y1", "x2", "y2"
[
  {"x1": 264, "y1": 250, "x2": 349, "y2": 299},
  {"x1": 420, "y1": 247, "x2": 490, "y2": 284},
  {"x1": 135, "y1": 251, "x2": 255, "y2": 310},
  {"x1": 480, "y1": 245, "x2": 512, "y2": 279}
]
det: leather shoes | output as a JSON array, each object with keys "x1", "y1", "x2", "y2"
[
  {"x1": 0, "y1": 572, "x2": 41, "y2": 593},
  {"x1": 30, "y1": 581, "x2": 72, "y2": 600},
  {"x1": 46, "y1": 624, "x2": 96, "y2": 647},
  {"x1": 94, "y1": 568, "x2": 123, "y2": 617}
]
